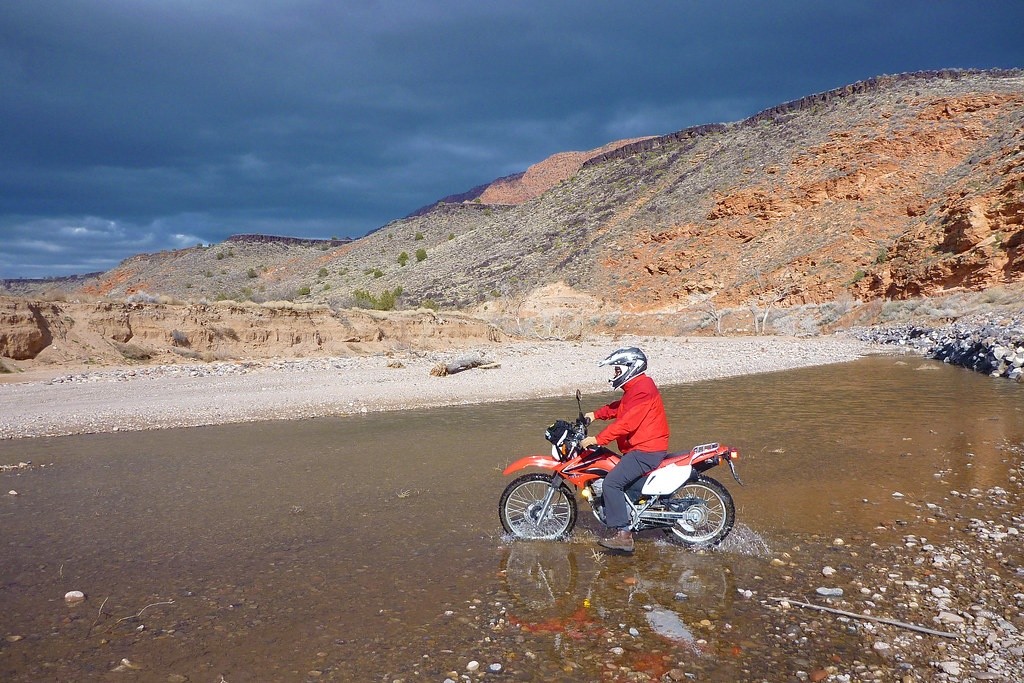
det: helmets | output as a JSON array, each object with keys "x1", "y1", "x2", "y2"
[{"x1": 598, "y1": 346, "x2": 647, "y2": 391}]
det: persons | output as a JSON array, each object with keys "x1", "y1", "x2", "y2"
[{"x1": 580, "y1": 347, "x2": 670, "y2": 551}]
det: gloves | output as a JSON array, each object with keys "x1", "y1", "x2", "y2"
[
  {"x1": 581, "y1": 436, "x2": 597, "y2": 450},
  {"x1": 583, "y1": 412, "x2": 595, "y2": 423}
]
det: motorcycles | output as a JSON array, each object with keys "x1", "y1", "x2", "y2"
[
  {"x1": 498, "y1": 389, "x2": 745, "y2": 550},
  {"x1": 494, "y1": 540, "x2": 736, "y2": 683}
]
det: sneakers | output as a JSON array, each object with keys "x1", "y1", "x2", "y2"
[{"x1": 598, "y1": 529, "x2": 634, "y2": 551}]
